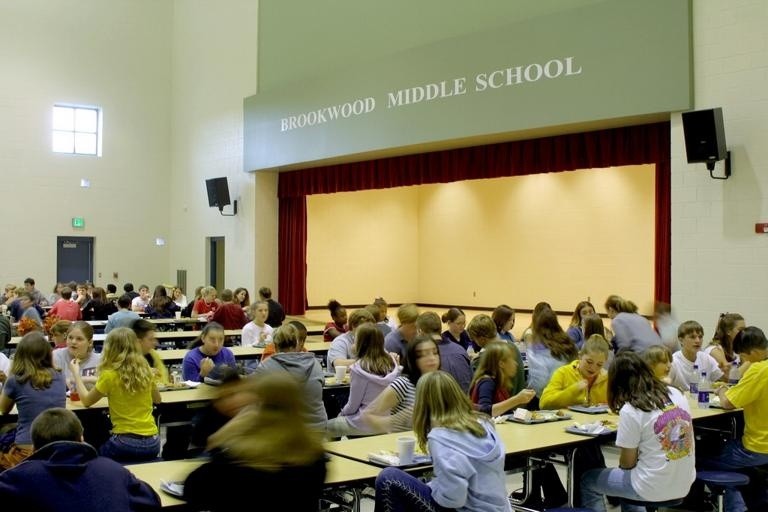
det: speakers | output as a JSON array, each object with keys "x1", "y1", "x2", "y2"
[
  {"x1": 681, "y1": 107, "x2": 728, "y2": 164},
  {"x1": 205, "y1": 177, "x2": 231, "y2": 207}
]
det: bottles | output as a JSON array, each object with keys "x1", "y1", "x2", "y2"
[
  {"x1": 690, "y1": 365, "x2": 700, "y2": 400},
  {"x1": 729, "y1": 362, "x2": 739, "y2": 386},
  {"x1": 519, "y1": 339, "x2": 526, "y2": 362},
  {"x1": 69, "y1": 372, "x2": 80, "y2": 400},
  {"x1": 698, "y1": 372, "x2": 710, "y2": 409}
]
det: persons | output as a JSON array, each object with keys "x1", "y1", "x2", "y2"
[
  {"x1": 0, "y1": 277, "x2": 332, "y2": 511},
  {"x1": 317, "y1": 294, "x2": 768, "y2": 512}
]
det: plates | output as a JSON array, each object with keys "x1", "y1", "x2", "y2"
[
  {"x1": 369, "y1": 454, "x2": 432, "y2": 469},
  {"x1": 158, "y1": 380, "x2": 201, "y2": 391},
  {"x1": 503, "y1": 403, "x2": 618, "y2": 438}
]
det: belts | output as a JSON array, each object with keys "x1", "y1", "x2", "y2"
[{"x1": 117, "y1": 433, "x2": 154, "y2": 439}]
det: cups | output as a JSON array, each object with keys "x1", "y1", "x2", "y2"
[
  {"x1": 175, "y1": 312, "x2": 181, "y2": 319},
  {"x1": 398, "y1": 436, "x2": 416, "y2": 466},
  {"x1": 335, "y1": 366, "x2": 346, "y2": 379}
]
